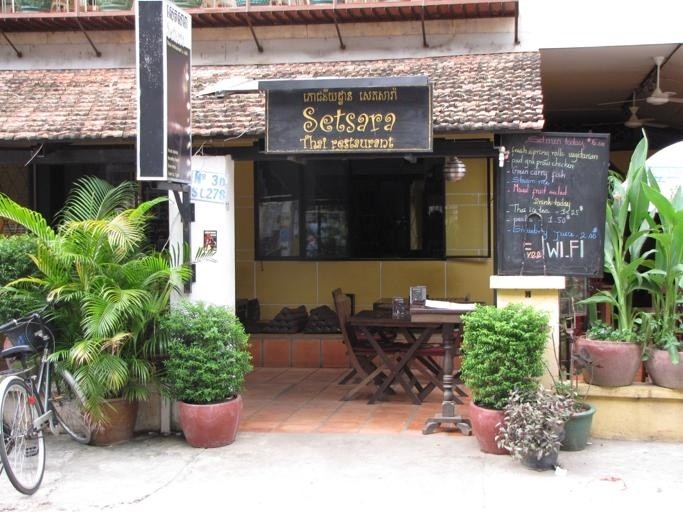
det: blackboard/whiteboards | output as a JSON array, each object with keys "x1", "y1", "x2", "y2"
[
  {"x1": 498, "y1": 132, "x2": 611, "y2": 276},
  {"x1": 266, "y1": 83, "x2": 433, "y2": 155}
]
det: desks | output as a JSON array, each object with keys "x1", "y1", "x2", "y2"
[{"x1": 348, "y1": 297, "x2": 465, "y2": 404}]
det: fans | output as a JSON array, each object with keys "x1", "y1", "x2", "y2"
[{"x1": 595, "y1": 57, "x2": 682, "y2": 130}]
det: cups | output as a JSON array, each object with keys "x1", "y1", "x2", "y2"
[
  {"x1": 410, "y1": 285, "x2": 427, "y2": 305},
  {"x1": 392, "y1": 296, "x2": 411, "y2": 321}
]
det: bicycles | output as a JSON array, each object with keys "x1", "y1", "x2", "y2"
[{"x1": 0, "y1": 296, "x2": 94, "y2": 498}]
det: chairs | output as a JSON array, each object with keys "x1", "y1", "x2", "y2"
[{"x1": 330, "y1": 288, "x2": 394, "y2": 398}]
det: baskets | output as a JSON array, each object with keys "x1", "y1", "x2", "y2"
[{"x1": 5, "y1": 317, "x2": 55, "y2": 357}]
